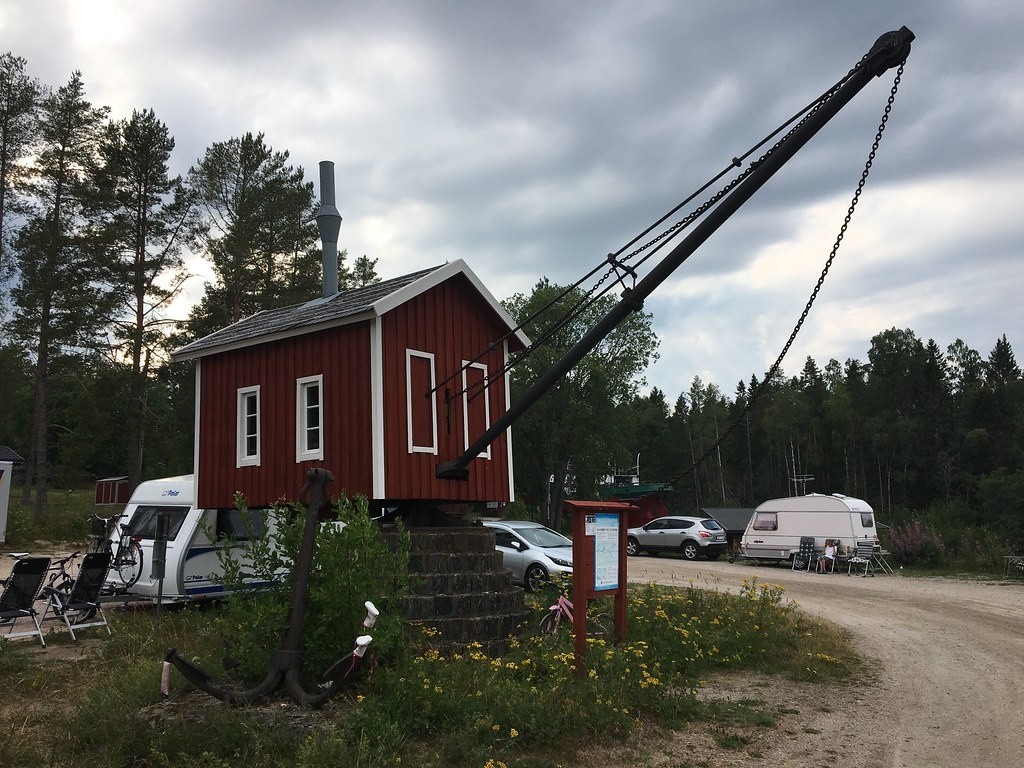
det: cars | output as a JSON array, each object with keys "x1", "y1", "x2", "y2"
[{"x1": 476, "y1": 519, "x2": 573, "y2": 595}]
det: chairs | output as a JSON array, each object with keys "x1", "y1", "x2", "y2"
[
  {"x1": 36, "y1": 551, "x2": 114, "y2": 642},
  {"x1": 847, "y1": 541, "x2": 875, "y2": 578},
  {"x1": 790, "y1": 536, "x2": 816, "y2": 572},
  {"x1": 0, "y1": 557, "x2": 51, "y2": 648},
  {"x1": 815, "y1": 538, "x2": 841, "y2": 573}
]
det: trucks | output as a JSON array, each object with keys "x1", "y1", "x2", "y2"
[
  {"x1": 740, "y1": 493, "x2": 879, "y2": 570},
  {"x1": 95, "y1": 469, "x2": 360, "y2": 607}
]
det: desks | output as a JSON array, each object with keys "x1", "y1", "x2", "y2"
[
  {"x1": 851, "y1": 552, "x2": 894, "y2": 574},
  {"x1": 1001, "y1": 556, "x2": 1024, "y2": 579}
]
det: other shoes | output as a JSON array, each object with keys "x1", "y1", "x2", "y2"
[{"x1": 818, "y1": 571, "x2": 827, "y2": 574}]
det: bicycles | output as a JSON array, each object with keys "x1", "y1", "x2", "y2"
[
  {"x1": 84, "y1": 513, "x2": 144, "y2": 587},
  {"x1": 0, "y1": 549, "x2": 94, "y2": 625}
]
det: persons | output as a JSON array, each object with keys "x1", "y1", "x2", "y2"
[{"x1": 817, "y1": 539, "x2": 838, "y2": 574}]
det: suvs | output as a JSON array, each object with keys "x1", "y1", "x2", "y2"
[{"x1": 627, "y1": 515, "x2": 729, "y2": 561}]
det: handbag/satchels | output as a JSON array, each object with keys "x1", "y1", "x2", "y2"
[{"x1": 92, "y1": 520, "x2": 111, "y2": 535}]
